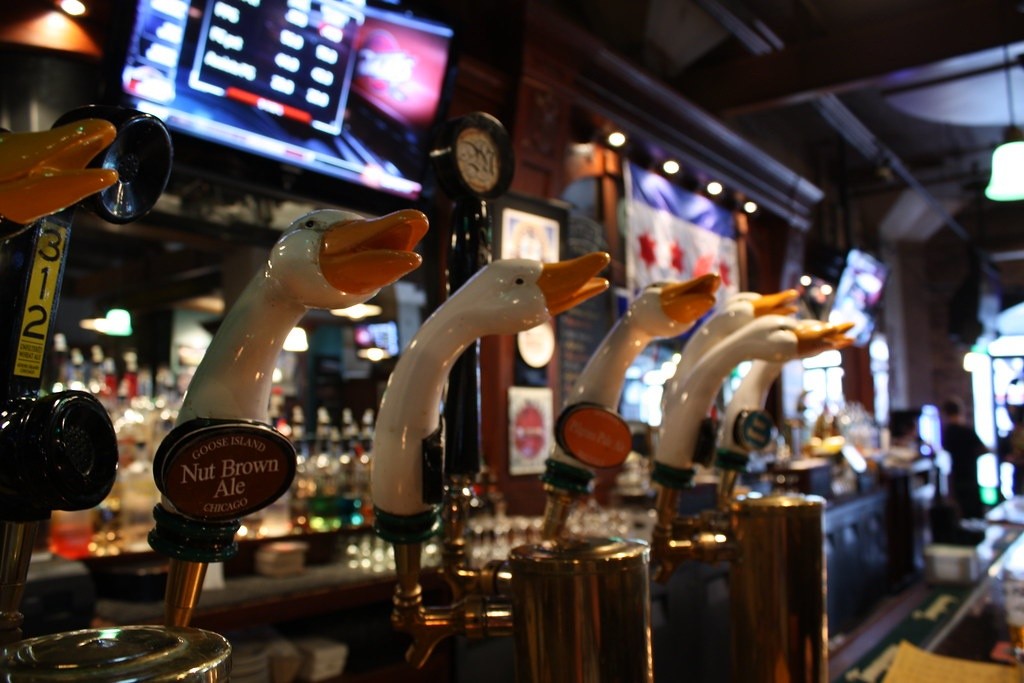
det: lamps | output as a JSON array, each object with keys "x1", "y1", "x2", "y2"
[{"x1": 984, "y1": 44, "x2": 1024, "y2": 202}]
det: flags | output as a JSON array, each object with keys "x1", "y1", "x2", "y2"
[{"x1": 620, "y1": 157, "x2": 742, "y2": 341}]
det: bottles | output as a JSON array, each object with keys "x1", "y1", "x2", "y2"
[
  {"x1": 107, "y1": 396, "x2": 177, "y2": 552},
  {"x1": 296, "y1": 453, "x2": 371, "y2": 498}
]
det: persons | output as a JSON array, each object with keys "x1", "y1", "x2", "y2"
[
  {"x1": 940, "y1": 397, "x2": 996, "y2": 520},
  {"x1": 996, "y1": 393, "x2": 1024, "y2": 497}
]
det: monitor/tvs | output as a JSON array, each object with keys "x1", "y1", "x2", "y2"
[
  {"x1": 100, "y1": 0, "x2": 461, "y2": 220},
  {"x1": 825, "y1": 245, "x2": 893, "y2": 348}
]
